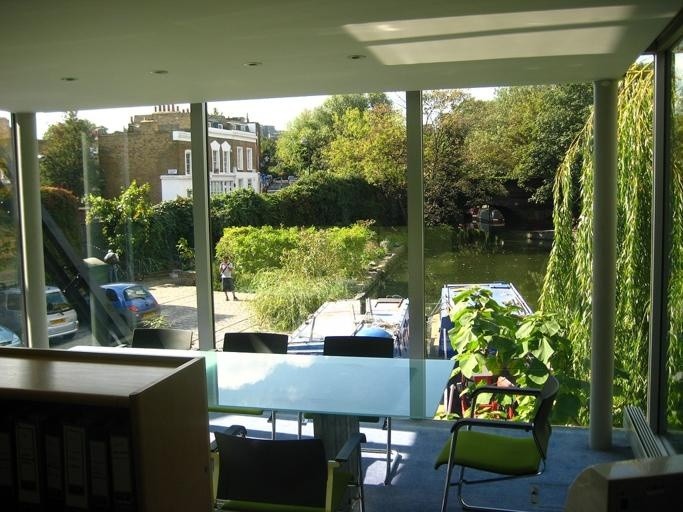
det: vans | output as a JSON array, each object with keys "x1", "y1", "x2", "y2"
[{"x1": 1, "y1": 284, "x2": 83, "y2": 342}]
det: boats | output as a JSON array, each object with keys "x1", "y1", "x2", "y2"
[{"x1": 455, "y1": 202, "x2": 507, "y2": 231}]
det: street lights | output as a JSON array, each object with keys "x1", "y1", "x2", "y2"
[
  {"x1": 263, "y1": 154, "x2": 269, "y2": 196},
  {"x1": 301, "y1": 140, "x2": 313, "y2": 177}
]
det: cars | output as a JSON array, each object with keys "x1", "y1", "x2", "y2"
[
  {"x1": 0, "y1": 321, "x2": 21, "y2": 350},
  {"x1": 91, "y1": 283, "x2": 161, "y2": 328},
  {"x1": 261, "y1": 172, "x2": 298, "y2": 195}
]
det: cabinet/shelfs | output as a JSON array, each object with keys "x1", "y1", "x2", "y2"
[{"x1": 0, "y1": 345, "x2": 216, "y2": 512}]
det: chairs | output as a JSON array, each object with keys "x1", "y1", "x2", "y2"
[
  {"x1": 207, "y1": 333, "x2": 288, "y2": 440},
  {"x1": 210, "y1": 426, "x2": 366, "y2": 512},
  {"x1": 298, "y1": 336, "x2": 401, "y2": 485},
  {"x1": 132, "y1": 328, "x2": 192, "y2": 349},
  {"x1": 434, "y1": 374, "x2": 560, "y2": 512}
]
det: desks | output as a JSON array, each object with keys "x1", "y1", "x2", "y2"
[{"x1": 69, "y1": 345, "x2": 455, "y2": 486}]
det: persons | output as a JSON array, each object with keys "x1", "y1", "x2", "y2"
[
  {"x1": 219, "y1": 256, "x2": 240, "y2": 301},
  {"x1": 103, "y1": 248, "x2": 120, "y2": 282}
]
